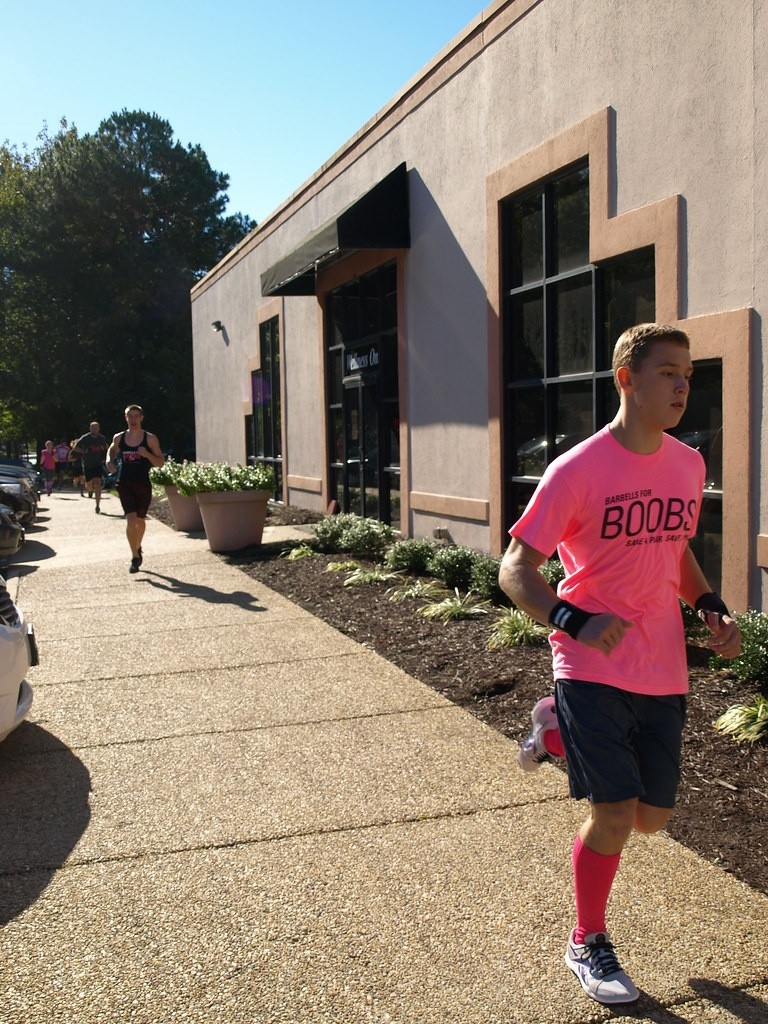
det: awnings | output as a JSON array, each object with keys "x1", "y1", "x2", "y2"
[{"x1": 260, "y1": 162, "x2": 410, "y2": 298}]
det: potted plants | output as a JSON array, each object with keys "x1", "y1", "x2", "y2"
[
  {"x1": 173, "y1": 460, "x2": 277, "y2": 552},
  {"x1": 148, "y1": 455, "x2": 213, "y2": 532}
]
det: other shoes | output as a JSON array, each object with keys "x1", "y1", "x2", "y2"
[
  {"x1": 73, "y1": 480, "x2": 78, "y2": 487},
  {"x1": 81, "y1": 493, "x2": 85, "y2": 497},
  {"x1": 89, "y1": 492, "x2": 92, "y2": 498},
  {"x1": 95, "y1": 506, "x2": 100, "y2": 513},
  {"x1": 47, "y1": 488, "x2": 52, "y2": 497}
]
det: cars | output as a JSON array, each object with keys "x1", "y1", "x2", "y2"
[
  {"x1": 0, "y1": 575, "x2": 38, "y2": 743},
  {"x1": 515, "y1": 432, "x2": 590, "y2": 478},
  {"x1": 0, "y1": 456, "x2": 42, "y2": 528},
  {"x1": 0, "y1": 503, "x2": 26, "y2": 559}
]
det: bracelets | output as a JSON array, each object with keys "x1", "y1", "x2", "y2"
[
  {"x1": 695, "y1": 591, "x2": 732, "y2": 625},
  {"x1": 548, "y1": 599, "x2": 597, "y2": 639}
]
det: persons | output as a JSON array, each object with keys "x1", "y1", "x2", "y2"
[
  {"x1": 65, "y1": 422, "x2": 109, "y2": 514},
  {"x1": 497, "y1": 321, "x2": 742, "y2": 1006},
  {"x1": 39, "y1": 440, "x2": 59, "y2": 496},
  {"x1": 107, "y1": 405, "x2": 165, "y2": 573}
]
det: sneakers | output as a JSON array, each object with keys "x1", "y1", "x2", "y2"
[
  {"x1": 130, "y1": 557, "x2": 139, "y2": 574},
  {"x1": 518, "y1": 697, "x2": 560, "y2": 772},
  {"x1": 137, "y1": 547, "x2": 144, "y2": 566},
  {"x1": 564, "y1": 926, "x2": 639, "y2": 1004}
]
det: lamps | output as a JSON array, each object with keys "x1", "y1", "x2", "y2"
[{"x1": 211, "y1": 320, "x2": 225, "y2": 333}]
